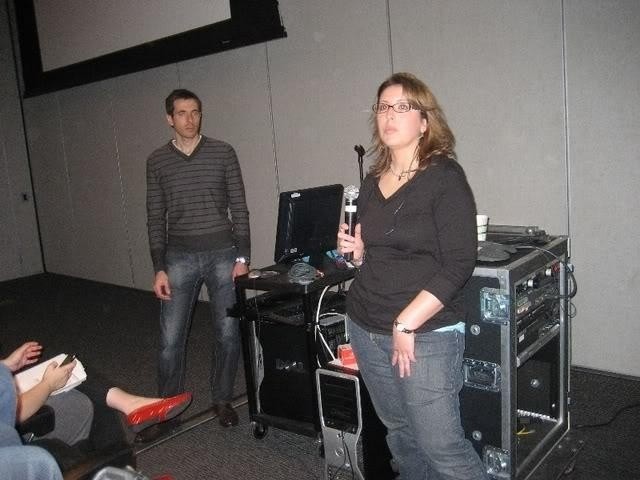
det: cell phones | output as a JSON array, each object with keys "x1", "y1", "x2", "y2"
[{"x1": 59, "y1": 351, "x2": 79, "y2": 367}]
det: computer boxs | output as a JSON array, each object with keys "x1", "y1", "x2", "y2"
[{"x1": 315, "y1": 360, "x2": 399, "y2": 480}]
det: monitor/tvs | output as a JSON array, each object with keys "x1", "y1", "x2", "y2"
[{"x1": 274, "y1": 183, "x2": 343, "y2": 268}]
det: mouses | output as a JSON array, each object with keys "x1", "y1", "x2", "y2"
[{"x1": 249, "y1": 270, "x2": 261, "y2": 280}]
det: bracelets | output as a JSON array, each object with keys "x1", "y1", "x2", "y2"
[{"x1": 235, "y1": 256, "x2": 249, "y2": 265}]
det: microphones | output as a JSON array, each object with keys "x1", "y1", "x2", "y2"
[{"x1": 343, "y1": 185, "x2": 359, "y2": 261}]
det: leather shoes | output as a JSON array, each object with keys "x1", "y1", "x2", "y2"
[
  {"x1": 133, "y1": 418, "x2": 177, "y2": 445},
  {"x1": 124, "y1": 391, "x2": 193, "y2": 433},
  {"x1": 213, "y1": 403, "x2": 239, "y2": 428}
]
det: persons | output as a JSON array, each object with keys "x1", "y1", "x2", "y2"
[
  {"x1": 0, "y1": 339, "x2": 193, "y2": 479},
  {"x1": 336, "y1": 71, "x2": 485, "y2": 479},
  {"x1": 134, "y1": 88, "x2": 250, "y2": 445}
]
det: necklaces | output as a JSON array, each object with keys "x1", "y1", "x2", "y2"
[{"x1": 391, "y1": 167, "x2": 401, "y2": 181}]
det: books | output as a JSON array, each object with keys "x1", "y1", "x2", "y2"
[{"x1": 12, "y1": 353, "x2": 87, "y2": 395}]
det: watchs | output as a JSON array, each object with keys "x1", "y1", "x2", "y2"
[{"x1": 393, "y1": 319, "x2": 412, "y2": 334}]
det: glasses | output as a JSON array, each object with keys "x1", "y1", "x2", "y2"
[{"x1": 372, "y1": 102, "x2": 423, "y2": 114}]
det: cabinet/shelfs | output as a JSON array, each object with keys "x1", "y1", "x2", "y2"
[{"x1": 233, "y1": 253, "x2": 358, "y2": 456}]
